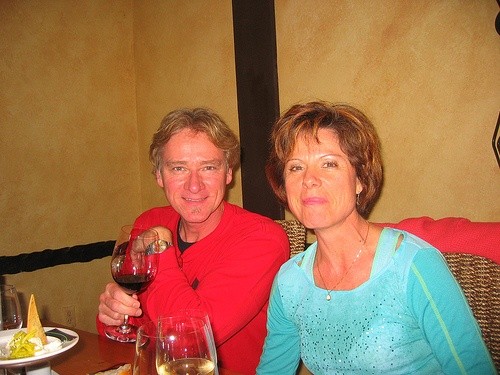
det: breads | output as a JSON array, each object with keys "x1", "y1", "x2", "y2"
[{"x1": 96, "y1": 364, "x2": 133, "y2": 375}]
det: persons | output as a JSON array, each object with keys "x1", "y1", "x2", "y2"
[
  {"x1": 256, "y1": 96, "x2": 498, "y2": 375},
  {"x1": 96, "y1": 106, "x2": 290, "y2": 375}
]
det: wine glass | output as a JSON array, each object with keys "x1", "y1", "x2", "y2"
[{"x1": 104, "y1": 225, "x2": 160, "y2": 343}]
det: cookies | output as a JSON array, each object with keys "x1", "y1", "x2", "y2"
[{"x1": 27, "y1": 294, "x2": 49, "y2": 345}]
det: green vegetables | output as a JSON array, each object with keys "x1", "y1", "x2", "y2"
[{"x1": 8, "y1": 330, "x2": 43, "y2": 360}]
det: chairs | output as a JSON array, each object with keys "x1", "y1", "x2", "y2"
[{"x1": 368, "y1": 217, "x2": 500, "y2": 375}]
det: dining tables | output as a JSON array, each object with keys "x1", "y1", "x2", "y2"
[{"x1": 0, "y1": 319, "x2": 245, "y2": 375}]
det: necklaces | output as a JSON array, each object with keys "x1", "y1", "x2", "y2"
[{"x1": 316, "y1": 221, "x2": 369, "y2": 302}]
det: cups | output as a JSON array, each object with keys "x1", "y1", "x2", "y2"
[
  {"x1": 132, "y1": 318, "x2": 217, "y2": 375},
  {"x1": 155, "y1": 309, "x2": 218, "y2": 375},
  {"x1": 0, "y1": 285, "x2": 23, "y2": 331}
]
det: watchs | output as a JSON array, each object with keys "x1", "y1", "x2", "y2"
[{"x1": 145, "y1": 239, "x2": 173, "y2": 255}]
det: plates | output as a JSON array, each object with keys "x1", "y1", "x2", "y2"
[{"x1": 0, "y1": 327, "x2": 79, "y2": 368}]
